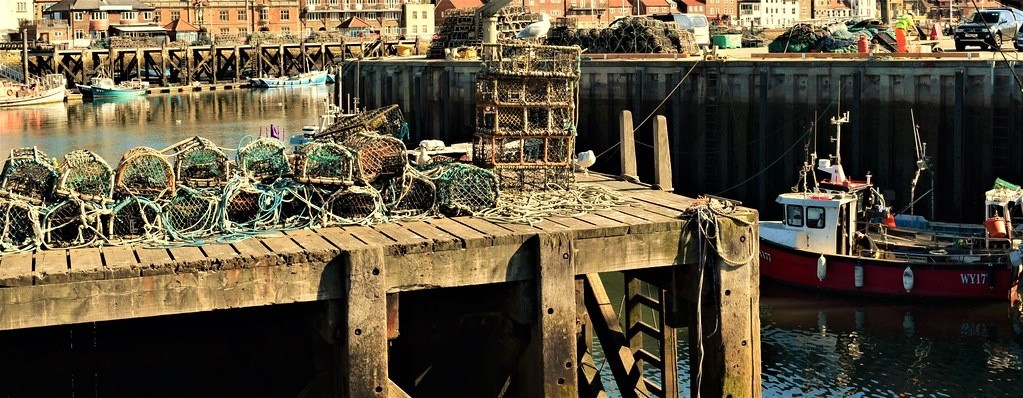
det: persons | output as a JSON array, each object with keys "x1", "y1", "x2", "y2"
[{"x1": 897, "y1": 12, "x2": 915, "y2": 30}]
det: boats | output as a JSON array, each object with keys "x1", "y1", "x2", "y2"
[
  {"x1": 757, "y1": 79, "x2": 1023, "y2": 303},
  {"x1": 0, "y1": 74, "x2": 67, "y2": 107},
  {"x1": 289, "y1": 70, "x2": 348, "y2": 154},
  {"x1": 74, "y1": 58, "x2": 148, "y2": 99},
  {"x1": 246, "y1": 69, "x2": 329, "y2": 87}
]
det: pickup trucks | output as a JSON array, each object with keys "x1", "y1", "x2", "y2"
[{"x1": 954, "y1": 9, "x2": 1023, "y2": 51}]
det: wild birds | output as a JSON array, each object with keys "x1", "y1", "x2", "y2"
[
  {"x1": 578, "y1": 149, "x2": 597, "y2": 179},
  {"x1": 418, "y1": 143, "x2": 433, "y2": 166},
  {"x1": 517, "y1": 13, "x2": 555, "y2": 45}
]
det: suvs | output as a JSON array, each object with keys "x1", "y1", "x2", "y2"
[{"x1": 609, "y1": 14, "x2": 695, "y2": 35}]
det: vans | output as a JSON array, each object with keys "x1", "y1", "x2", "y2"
[{"x1": 684, "y1": 13, "x2": 710, "y2": 48}]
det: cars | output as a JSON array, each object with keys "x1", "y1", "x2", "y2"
[{"x1": 1015, "y1": 23, "x2": 1023, "y2": 50}]
